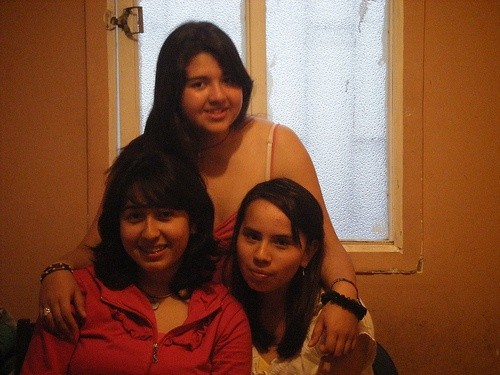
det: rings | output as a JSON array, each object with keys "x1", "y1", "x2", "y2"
[{"x1": 39, "y1": 304, "x2": 51, "y2": 317}]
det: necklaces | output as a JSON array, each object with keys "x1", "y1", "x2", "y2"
[{"x1": 138, "y1": 278, "x2": 176, "y2": 310}]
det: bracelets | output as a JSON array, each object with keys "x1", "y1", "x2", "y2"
[
  {"x1": 321, "y1": 288, "x2": 368, "y2": 321},
  {"x1": 330, "y1": 277, "x2": 359, "y2": 301},
  {"x1": 39, "y1": 261, "x2": 74, "y2": 288}
]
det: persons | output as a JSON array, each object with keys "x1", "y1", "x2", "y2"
[
  {"x1": 223, "y1": 175, "x2": 374, "y2": 375},
  {"x1": 18, "y1": 144, "x2": 253, "y2": 375},
  {"x1": 38, "y1": 20, "x2": 361, "y2": 360}
]
